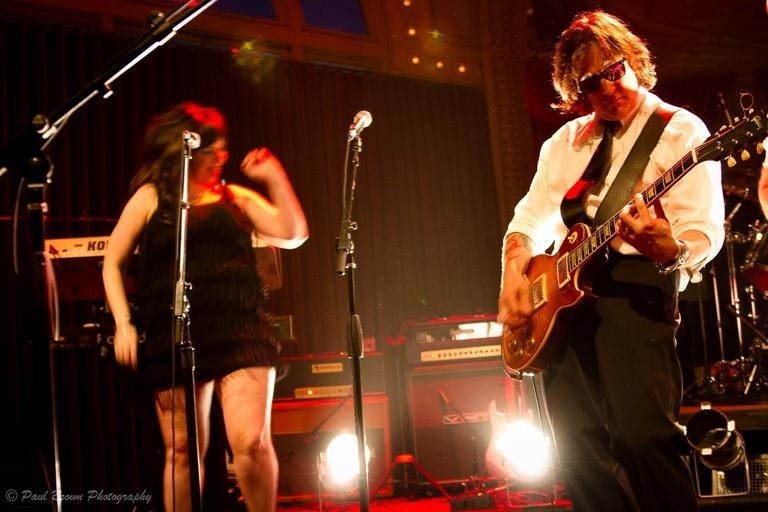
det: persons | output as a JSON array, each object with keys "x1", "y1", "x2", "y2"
[
  {"x1": 496, "y1": 6, "x2": 728, "y2": 512},
  {"x1": 101, "y1": 100, "x2": 312, "y2": 512}
]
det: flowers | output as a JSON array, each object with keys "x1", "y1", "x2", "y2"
[
  {"x1": 346, "y1": 110, "x2": 373, "y2": 142},
  {"x1": 183, "y1": 129, "x2": 201, "y2": 149}
]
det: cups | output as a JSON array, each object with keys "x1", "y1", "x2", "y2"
[{"x1": 578, "y1": 56, "x2": 626, "y2": 91}]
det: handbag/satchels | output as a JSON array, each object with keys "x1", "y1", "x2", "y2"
[{"x1": 655, "y1": 238, "x2": 691, "y2": 277}]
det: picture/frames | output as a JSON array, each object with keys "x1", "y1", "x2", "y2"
[{"x1": 271, "y1": 356, "x2": 521, "y2": 503}]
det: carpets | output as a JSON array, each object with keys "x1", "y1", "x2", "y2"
[{"x1": 560, "y1": 120, "x2": 617, "y2": 228}]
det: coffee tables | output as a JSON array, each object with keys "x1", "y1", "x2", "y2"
[{"x1": 722, "y1": 167, "x2": 758, "y2": 202}]
